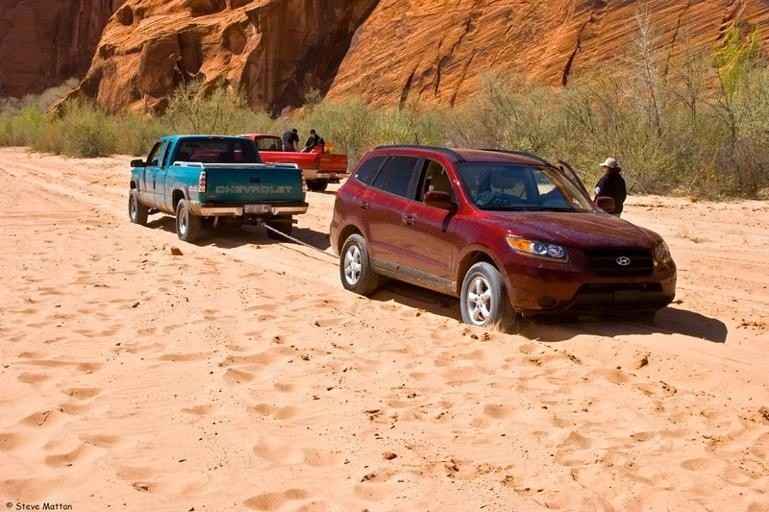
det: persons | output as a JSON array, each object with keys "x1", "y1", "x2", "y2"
[
  {"x1": 283, "y1": 128, "x2": 299, "y2": 151},
  {"x1": 301, "y1": 129, "x2": 325, "y2": 153},
  {"x1": 594, "y1": 157, "x2": 626, "y2": 218}
]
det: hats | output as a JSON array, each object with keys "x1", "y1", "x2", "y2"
[{"x1": 600, "y1": 158, "x2": 616, "y2": 168}]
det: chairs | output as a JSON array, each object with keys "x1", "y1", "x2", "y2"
[
  {"x1": 490, "y1": 173, "x2": 527, "y2": 206},
  {"x1": 269, "y1": 143, "x2": 276, "y2": 150},
  {"x1": 217, "y1": 150, "x2": 236, "y2": 162},
  {"x1": 173, "y1": 150, "x2": 189, "y2": 161},
  {"x1": 430, "y1": 174, "x2": 466, "y2": 198}
]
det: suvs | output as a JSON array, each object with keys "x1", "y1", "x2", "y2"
[{"x1": 331, "y1": 140, "x2": 679, "y2": 339}]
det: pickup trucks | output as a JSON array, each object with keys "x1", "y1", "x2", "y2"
[
  {"x1": 126, "y1": 134, "x2": 309, "y2": 243},
  {"x1": 192, "y1": 131, "x2": 350, "y2": 193}
]
